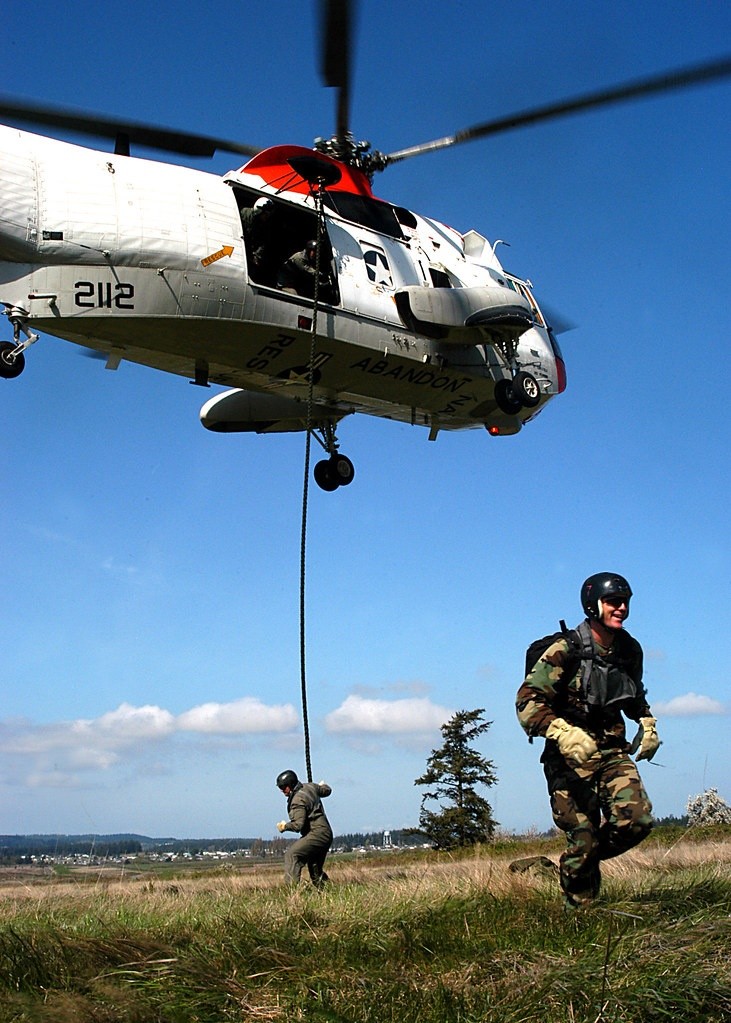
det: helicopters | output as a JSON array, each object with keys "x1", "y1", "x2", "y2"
[{"x1": 0, "y1": 0, "x2": 731, "y2": 493}]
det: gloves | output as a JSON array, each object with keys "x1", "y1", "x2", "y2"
[
  {"x1": 319, "y1": 780, "x2": 324, "y2": 786},
  {"x1": 277, "y1": 820, "x2": 286, "y2": 833},
  {"x1": 629, "y1": 717, "x2": 660, "y2": 761},
  {"x1": 546, "y1": 717, "x2": 598, "y2": 765}
]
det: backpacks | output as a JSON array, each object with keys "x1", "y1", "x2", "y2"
[{"x1": 526, "y1": 620, "x2": 584, "y2": 692}]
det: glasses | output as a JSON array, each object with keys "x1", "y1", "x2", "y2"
[{"x1": 605, "y1": 597, "x2": 628, "y2": 607}]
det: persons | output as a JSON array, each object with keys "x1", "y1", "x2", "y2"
[
  {"x1": 240, "y1": 197, "x2": 274, "y2": 273},
  {"x1": 276, "y1": 770, "x2": 334, "y2": 889},
  {"x1": 516, "y1": 572, "x2": 661, "y2": 911},
  {"x1": 285, "y1": 239, "x2": 328, "y2": 296}
]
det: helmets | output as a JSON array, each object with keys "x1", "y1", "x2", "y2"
[
  {"x1": 307, "y1": 240, "x2": 321, "y2": 252},
  {"x1": 253, "y1": 197, "x2": 274, "y2": 216},
  {"x1": 581, "y1": 572, "x2": 633, "y2": 620},
  {"x1": 277, "y1": 770, "x2": 297, "y2": 790}
]
